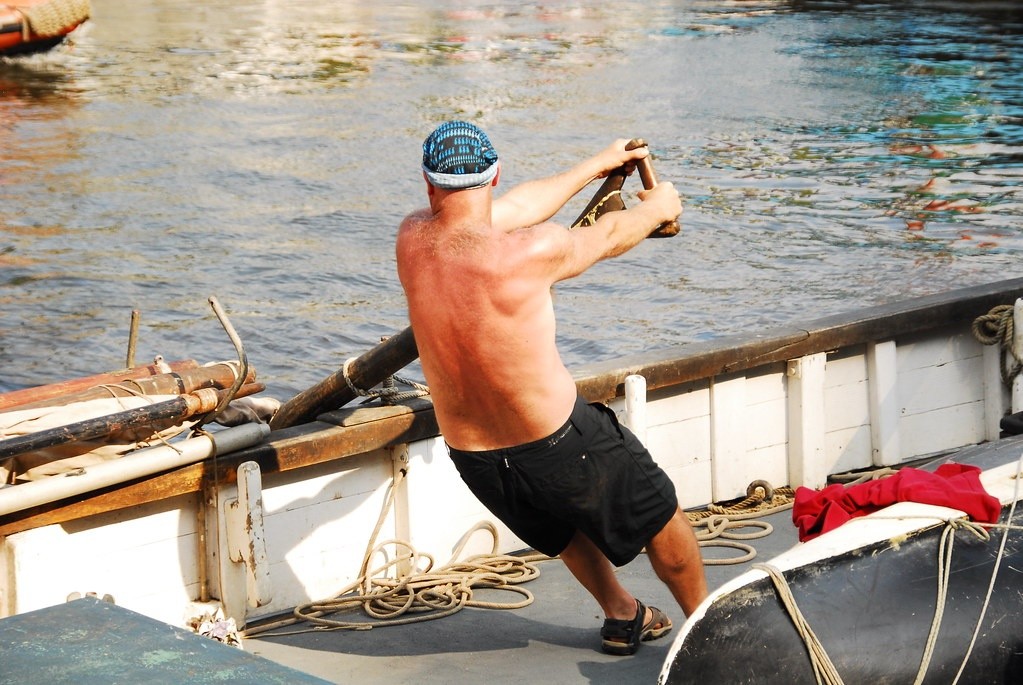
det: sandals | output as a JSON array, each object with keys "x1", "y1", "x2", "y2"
[{"x1": 600, "y1": 598, "x2": 672, "y2": 655}]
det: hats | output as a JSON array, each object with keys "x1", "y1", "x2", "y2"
[{"x1": 421, "y1": 120, "x2": 499, "y2": 191}]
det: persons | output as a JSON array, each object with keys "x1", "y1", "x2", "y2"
[{"x1": 395, "y1": 120, "x2": 708, "y2": 655}]
